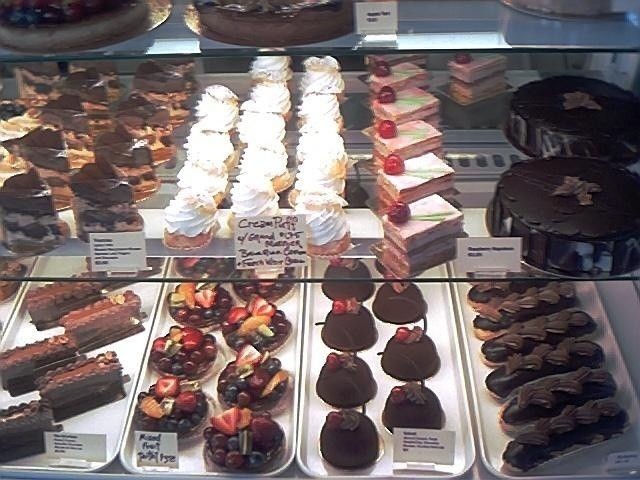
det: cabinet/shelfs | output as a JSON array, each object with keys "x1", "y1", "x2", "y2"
[{"x1": 0, "y1": 0, "x2": 640, "y2": 480}]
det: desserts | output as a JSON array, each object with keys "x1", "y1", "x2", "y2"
[
  {"x1": 0, "y1": 54, "x2": 199, "y2": 258},
  {"x1": 162, "y1": 49, "x2": 351, "y2": 257}
]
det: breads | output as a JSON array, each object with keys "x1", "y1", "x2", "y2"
[
  {"x1": 0, "y1": 257, "x2": 164, "y2": 464},
  {"x1": 468, "y1": 280, "x2": 629, "y2": 473}
]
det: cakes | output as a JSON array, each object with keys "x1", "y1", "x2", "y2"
[
  {"x1": 372, "y1": 282, "x2": 428, "y2": 324},
  {"x1": 368, "y1": 61, "x2": 465, "y2": 278},
  {"x1": 321, "y1": 296, "x2": 378, "y2": 351},
  {"x1": 321, "y1": 259, "x2": 375, "y2": 302},
  {"x1": 436, "y1": 51, "x2": 518, "y2": 106},
  {"x1": 134, "y1": 257, "x2": 299, "y2": 472},
  {"x1": 381, "y1": 384, "x2": 447, "y2": 435},
  {"x1": 318, "y1": 411, "x2": 384, "y2": 470},
  {"x1": 316, "y1": 351, "x2": 378, "y2": 408},
  {"x1": 504, "y1": 76, "x2": 640, "y2": 167},
  {"x1": 381, "y1": 326, "x2": 441, "y2": 381},
  {"x1": 0, "y1": 0, "x2": 148, "y2": 53},
  {"x1": 486, "y1": 157, "x2": 640, "y2": 278},
  {"x1": 193, "y1": 1, "x2": 354, "y2": 46}
]
